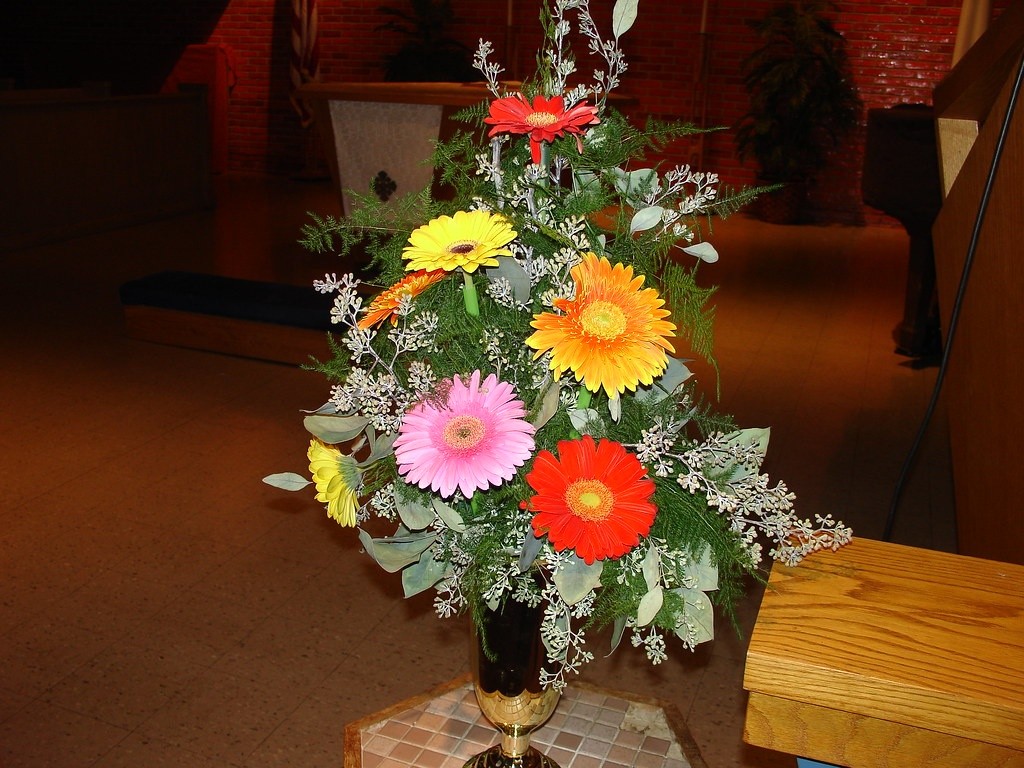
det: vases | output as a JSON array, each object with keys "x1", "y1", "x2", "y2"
[{"x1": 459, "y1": 568, "x2": 561, "y2": 768}]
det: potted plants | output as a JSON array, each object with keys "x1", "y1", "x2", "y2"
[{"x1": 728, "y1": 0, "x2": 854, "y2": 221}]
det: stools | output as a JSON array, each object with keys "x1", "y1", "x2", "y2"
[{"x1": 743, "y1": 529, "x2": 1024, "y2": 768}]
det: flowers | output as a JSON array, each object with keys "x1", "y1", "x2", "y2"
[{"x1": 297, "y1": 0, "x2": 855, "y2": 690}]
